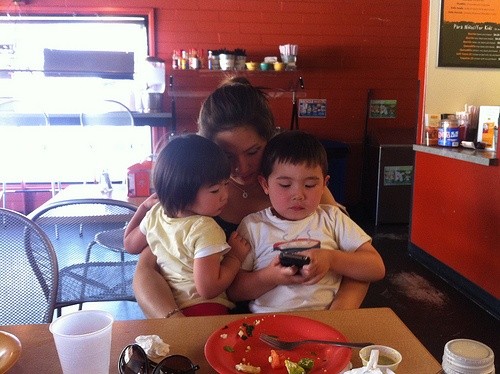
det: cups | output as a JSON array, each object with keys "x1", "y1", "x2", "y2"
[{"x1": 48, "y1": 311, "x2": 114, "y2": 374}]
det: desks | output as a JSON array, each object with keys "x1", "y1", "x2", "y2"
[
  {"x1": 25, "y1": 184, "x2": 147, "y2": 223},
  {"x1": 0, "y1": 306, "x2": 444, "y2": 374}
]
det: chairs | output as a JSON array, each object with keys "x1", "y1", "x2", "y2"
[
  {"x1": 0, "y1": 209, "x2": 59, "y2": 324},
  {"x1": 79, "y1": 227, "x2": 127, "y2": 311},
  {"x1": 24, "y1": 197, "x2": 137, "y2": 317},
  {"x1": 0, "y1": 96, "x2": 134, "y2": 239}
]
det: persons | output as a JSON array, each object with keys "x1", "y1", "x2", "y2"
[
  {"x1": 227, "y1": 130, "x2": 386, "y2": 315},
  {"x1": 124, "y1": 134, "x2": 252, "y2": 316},
  {"x1": 132, "y1": 77, "x2": 368, "y2": 319}
]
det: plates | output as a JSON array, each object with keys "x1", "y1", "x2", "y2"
[
  {"x1": 0, "y1": 330, "x2": 23, "y2": 374},
  {"x1": 203, "y1": 315, "x2": 352, "y2": 373}
]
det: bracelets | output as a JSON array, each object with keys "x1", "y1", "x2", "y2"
[{"x1": 164, "y1": 308, "x2": 181, "y2": 319}]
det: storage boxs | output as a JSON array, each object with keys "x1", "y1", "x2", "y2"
[{"x1": 127, "y1": 161, "x2": 151, "y2": 197}]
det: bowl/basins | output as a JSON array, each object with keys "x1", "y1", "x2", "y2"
[
  {"x1": 245, "y1": 61, "x2": 258, "y2": 71},
  {"x1": 359, "y1": 344, "x2": 403, "y2": 371},
  {"x1": 272, "y1": 63, "x2": 286, "y2": 71},
  {"x1": 259, "y1": 63, "x2": 271, "y2": 71}
]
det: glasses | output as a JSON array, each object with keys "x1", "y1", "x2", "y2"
[{"x1": 117, "y1": 344, "x2": 199, "y2": 374}]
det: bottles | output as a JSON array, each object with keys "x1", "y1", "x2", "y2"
[
  {"x1": 434, "y1": 339, "x2": 495, "y2": 374},
  {"x1": 437, "y1": 119, "x2": 461, "y2": 147},
  {"x1": 170, "y1": 46, "x2": 219, "y2": 70}
]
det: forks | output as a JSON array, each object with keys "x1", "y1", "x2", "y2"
[{"x1": 258, "y1": 333, "x2": 374, "y2": 350}]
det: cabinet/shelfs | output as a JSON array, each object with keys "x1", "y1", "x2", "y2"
[{"x1": 165, "y1": 67, "x2": 306, "y2": 99}]
